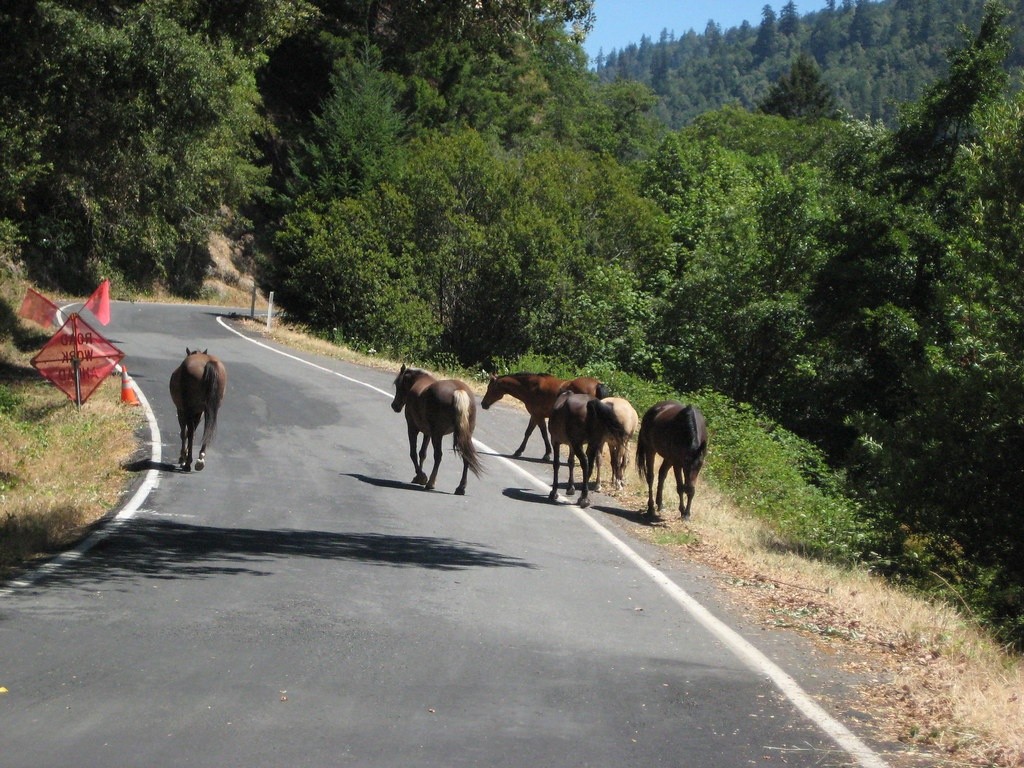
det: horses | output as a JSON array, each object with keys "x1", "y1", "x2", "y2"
[
  {"x1": 391, "y1": 363, "x2": 488, "y2": 496},
  {"x1": 545, "y1": 388, "x2": 639, "y2": 509},
  {"x1": 635, "y1": 398, "x2": 708, "y2": 522},
  {"x1": 169, "y1": 347, "x2": 227, "y2": 471},
  {"x1": 481, "y1": 369, "x2": 610, "y2": 466}
]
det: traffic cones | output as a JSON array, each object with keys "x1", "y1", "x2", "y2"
[{"x1": 119, "y1": 363, "x2": 140, "y2": 407}]
